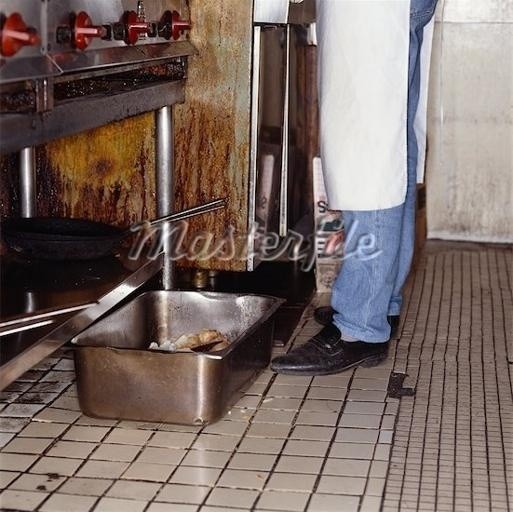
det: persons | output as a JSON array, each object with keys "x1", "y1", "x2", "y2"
[{"x1": 269, "y1": 0, "x2": 439, "y2": 376}]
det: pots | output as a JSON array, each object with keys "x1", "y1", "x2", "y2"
[{"x1": 1, "y1": 192, "x2": 227, "y2": 262}]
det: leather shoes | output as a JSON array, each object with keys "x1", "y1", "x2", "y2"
[
  {"x1": 271, "y1": 324, "x2": 387, "y2": 373},
  {"x1": 314, "y1": 304, "x2": 400, "y2": 339}
]
